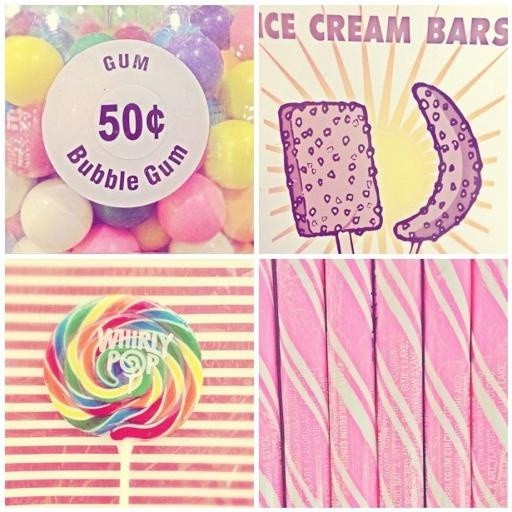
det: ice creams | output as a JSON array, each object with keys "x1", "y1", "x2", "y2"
[
  {"x1": 277, "y1": 100, "x2": 383, "y2": 254},
  {"x1": 391, "y1": 81, "x2": 483, "y2": 254}
]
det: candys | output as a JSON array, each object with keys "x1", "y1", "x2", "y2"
[
  {"x1": 4, "y1": 5, "x2": 254, "y2": 254},
  {"x1": 41, "y1": 293, "x2": 201, "y2": 505}
]
